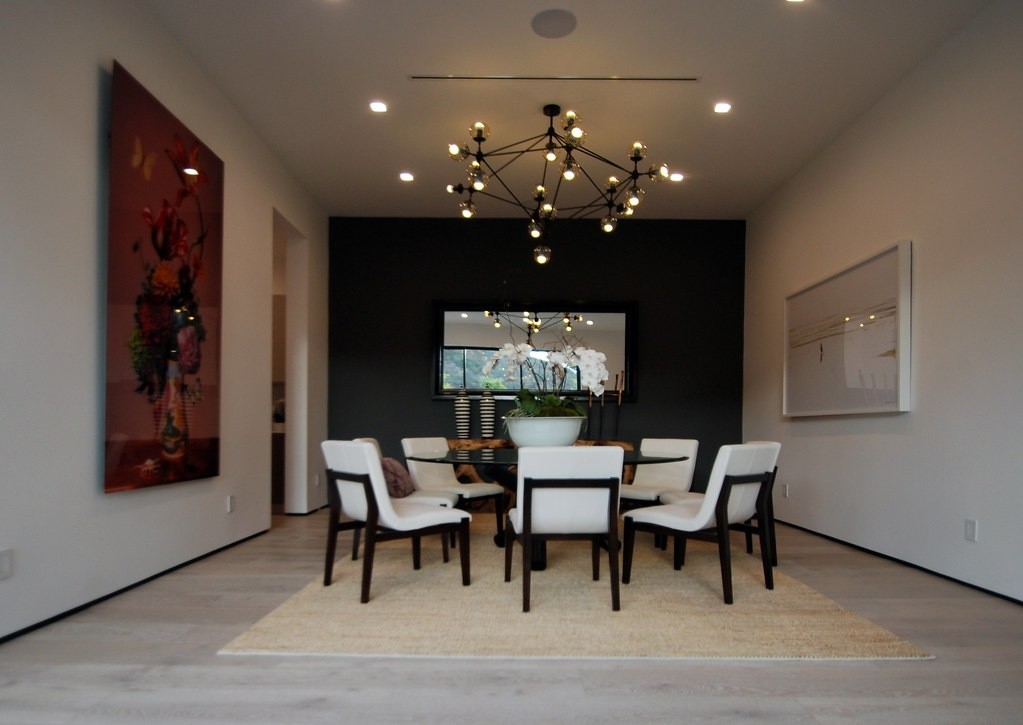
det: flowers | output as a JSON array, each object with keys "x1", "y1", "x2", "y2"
[
  {"x1": 483, "y1": 343, "x2": 608, "y2": 435},
  {"x1": 128, "y1": 134, "x2": 206, "y2": 404}
]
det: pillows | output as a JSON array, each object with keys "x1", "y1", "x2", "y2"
[{"x1": 380, "y1": 456, "x2": 415, "y2": 497}]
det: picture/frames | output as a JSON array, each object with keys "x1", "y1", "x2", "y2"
[{"x1": 781, "y1": 239, "x2": 911, "y2": 416}]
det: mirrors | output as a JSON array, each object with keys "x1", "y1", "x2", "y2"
[{"x1": 439, "y1": 306, "x2": 627, "y2": 395}]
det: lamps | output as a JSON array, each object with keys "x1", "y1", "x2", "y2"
[
  {"x1": 482, "y1": 310, "x2": 594, "y2": 345},
  {"x1": 445, "y1": 104, "x2": 671, "y2": 264}
]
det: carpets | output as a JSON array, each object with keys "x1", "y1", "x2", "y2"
[{"x1": 215, "y1": 510, "x2": 935, "y2": 658}]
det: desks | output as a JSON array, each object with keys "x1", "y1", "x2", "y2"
[
  {"x1": 446, "y1": 439, "x2": 633, "y2": 513},
  {"x1": 404, "y1": 447, "x2": 688, "y2": 571}
]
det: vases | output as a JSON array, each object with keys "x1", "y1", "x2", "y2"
[
  {"x1": 499, "y1": 415, "x2": 587, "y2": 452},
  {"x1": 160, "y1": 360, "x2": 188, "y2": 459}
]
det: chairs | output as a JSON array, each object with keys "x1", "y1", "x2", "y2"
[
  {"x1": 319, "y1": 438, "x2": 506, "y2": 605},
  {"x1": 504, "y1": 437, "x2": 782, "y2": 612}
]
